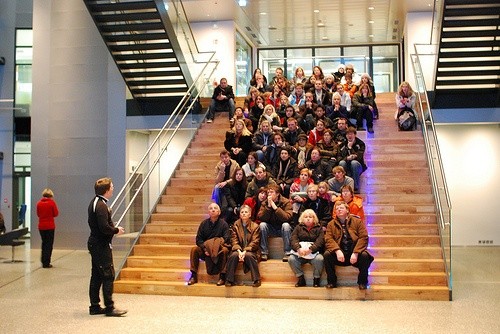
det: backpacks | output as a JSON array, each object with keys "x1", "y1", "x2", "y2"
[{"x1": 398, "y1": 105, "x2": 417, "y2": 132}]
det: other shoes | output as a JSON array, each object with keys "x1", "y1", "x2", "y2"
[
  {"x1": 313, "y1": 282, "x2": 320, "y2": 287},
  {"x1": 359, "y1": 283, "x2": 367, "y2": 290},
  {"x1": 368, "y1": 128, "x2": 374, "y2": 134},
  {"x1": 217, "y1": 278, "x2": 225, "y2": 286},
  {"x1": 225, "y1": 281, "x2": 233, "y2": 286},
  {"x1": 253, "y1": 280, "x2": 261, "y2": 287},
  {"x1": 89, "y1": 305, "x2": 106, "y2": 315},
  {"x1": 105, "y1": 308, "x2": 127, "y2": 317},
  {"x1": 43, "y1": 264, "x2": 53, "y2": 268},
  {"x1": 207, "y1": 119, "x2": 213, "y2": 123},
  {"x1": 327, "y1": 283, "x2": 337, "y2": 288},
  {"x1": 295, "y1": 279, "x2": 306, "y2": 287},
  {"x1": 261, "y1": 252, "x2": 268, "y2": 261},
  {"x1": 188, "y1": 277, "x2": 197, "y2": 285},
  {"x1": 282, "y1": 254, "x2": 289, "y2": 262}
]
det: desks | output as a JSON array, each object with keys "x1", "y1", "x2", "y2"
[{"x1": 116, "y1": 232, "x2": 139, "y2": 254}]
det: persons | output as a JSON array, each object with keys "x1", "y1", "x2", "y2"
[
  {"x1": 288, "y1": 209, "x2": 325, "y2": 287},
  {"x1": 225, "y1": 205, "x2": 262, "y2": 287},
  {"x1": 393, "y1": 81, "x2": 419, "y2": 131},
  {"x1": 242, "y1": 63, "x2": 379, "y2": 133},
  {"x1": 186, "y1": 203, "x2": 230, "y2": 286},
  {"x1": 37, "y1": 188, "x2": 58, "y2": 268},
  {"x1": 322, "y1": 200, "x2": 374, "y2": 290},
  {"x1": 211, "y1": 152, "x2": 365, "y2": 226},
  {"x1": 256, "y1": 185, "x2": 293, "y2": 263},
  {"x1": 206, "y1": 78, "x2": 235, "y2": 123},
  {"x1": 87, "y1": 177, "x2": 129, "y2": 316},
  {"x1": 223, "y1": 105, "x2": 368, "y2": 194}
]
